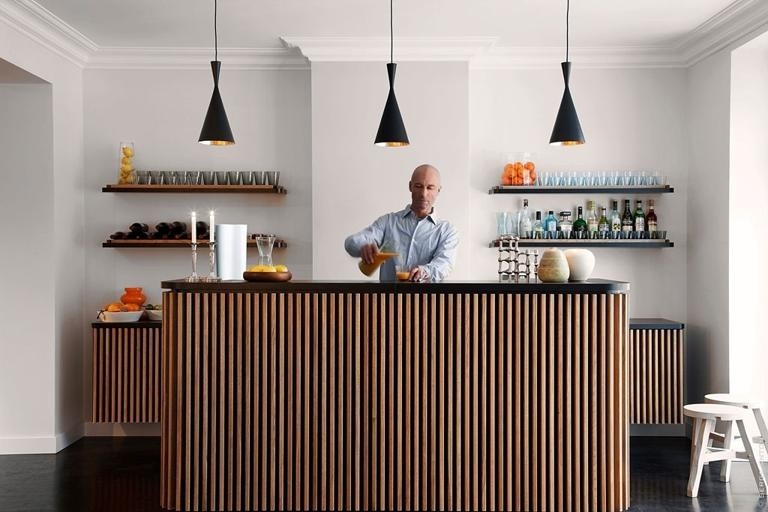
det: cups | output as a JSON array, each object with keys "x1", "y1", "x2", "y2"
[
  {"x1": 138, "y1": 170, "x2": 281, "y2": 186},
  {"x1": 358, "y1": 240, "x2": 401, "y2": 277},
  {"x1": 255, "y1": 237, "x2": 276, "y2": 265},
  {"x1": 536, "y1": 169, "x2": 668, "y2": 187},
  {"x1": 496, "y1": 210, "x2": 522, "y2": 237},
  {"x1": 525, "y1": 230, "x2": 667, "y2": 240},
  {"x1": 395, "y1": 265, "x2": 412, "y2": 281}
]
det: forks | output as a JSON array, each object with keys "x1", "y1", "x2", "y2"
[{"x1": 97, "y1": 308, "x2": 105, "y2": 318}]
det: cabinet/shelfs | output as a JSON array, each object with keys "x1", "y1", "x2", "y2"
[
  {"x1": 488, "y1": 183, "x2": 677, "y2": 249},
  {"x1": 100, "y1": 184, "x2": 289, "y2": 250}
]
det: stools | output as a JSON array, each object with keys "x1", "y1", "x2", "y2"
[{"x1": 682, "y1": 392, "x2": 768, "y2": 499}]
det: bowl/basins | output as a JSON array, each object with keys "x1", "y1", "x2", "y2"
[
  {"x1": 243, "y1": 272, "x2": 292, "y2": 281},
  {"x1": 146, "y1": 310, "x2": 162, "y2": 320},
  {"x1": 96, "y1": 310, "x2": 143, "y2": 322},
  {"x1": 495, "y1": 150, "x2": 542, "y2": 187}
]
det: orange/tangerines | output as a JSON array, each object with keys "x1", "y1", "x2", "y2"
[
  {"x1": 502, "y1": 160, "x2": 537, "y2": 185},
  {"x1": 122, "y1": 303, "x2": 133, "y2": 312},
  {"x1": 132, "y1": 302, "x2": 138, "y2": 311},
  {"x1": 105, "y1": 304, "x2": 120, "y2": 312}
]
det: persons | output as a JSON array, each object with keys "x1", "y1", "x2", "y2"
[{"x1": 344, "y1": 164, "x2": 461, "y2": 282}]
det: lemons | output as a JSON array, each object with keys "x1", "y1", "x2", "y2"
[
  {"x1": 263, "y1": 265, "x2": 276, "y2": 273},
  {"x1": 120, "y1": 165, "x2": 132, "y2": 172},
  {"x1": 250, "y1": 263, "x2": 265, "y2": 273},
  {"x1": 125, "y1": 174, "x2": 135, "y2": 184},
  {"x1": 123, "y1": 146, "x2": 134, "y2": 158},
  {"x1": 119, "y1": 178, "x2": 126, "y2": 184},
  {"x1": 120, "y1": 156, "x2": 129, "y2": 165},
  {"x1": 119, "y1": 171, "x2": 127, "y2": 179},
  {"x1": 274, "y1": 264, "x2": 289, "y2": 273}
]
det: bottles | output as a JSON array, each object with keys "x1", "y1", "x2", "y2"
[
  {"x1": 520, "y1": 197, "x2": 659, "y2": 238},
  {"x1": 119, "y1": 141, "x2": 138, "y2": 186},
  {"x1": 109, "y1": 220, "x2": 211, "y2": 240}
]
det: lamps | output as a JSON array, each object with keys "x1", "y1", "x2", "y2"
[
  {"x1": 195, "y1": 1, "x2": 236, "y2": 147},
  {"x1": 372, "y1": 1, "x2": 410, "y2": 148},
  {"x1": 547, "y1": 1, "x2": 586, "y2": 149}
]
